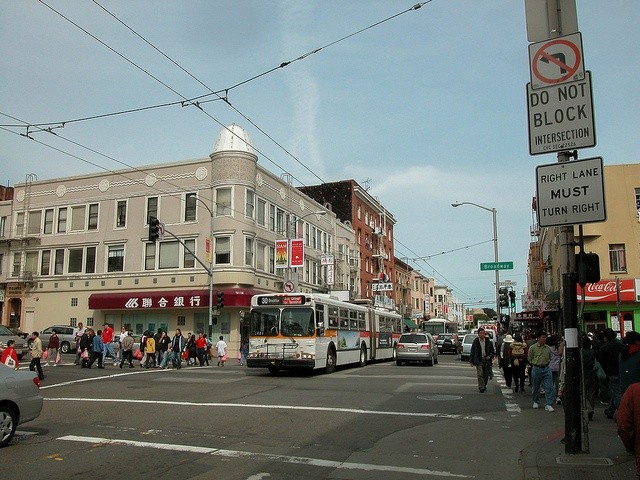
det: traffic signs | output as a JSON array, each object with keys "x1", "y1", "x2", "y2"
[{"x1": 480, "y1": 261, "x2": 513, "y2": 271}]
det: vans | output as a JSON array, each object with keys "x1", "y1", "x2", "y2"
[
  {"x1": 458, "y1": 330, "x2": 468, "y2": 341},
  {"x1": 27, "y1": 325, "x2": 79, "y2": 354},
  {"x1": 471, "y1": 329, "x2": 496, "y2": 353}
]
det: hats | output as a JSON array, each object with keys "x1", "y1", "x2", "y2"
[{"x1": 502, "y1": 334, "x2": 516, "y2": 343}]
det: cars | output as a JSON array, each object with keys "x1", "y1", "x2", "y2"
[
  {"x1": 0, "y1": 362, "x2": 43, "y2": 448},
  {"x1": 105, "y1": 334, "x2": 144, "y2": 357},
  {"x1": 6, "y1": 326, "x2": 29, "y2": 339}
]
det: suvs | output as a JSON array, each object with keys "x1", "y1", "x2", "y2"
[
  {"x1": 0, "y1": 325, "x2": 29, "y2": 359},
  {"x1": 396, "y1": 332, "x2": 438, "y2": 366},
  {"x1": 437, "y1": 333, "x2": 461, "y2": 355},
  {"x1": 460, "y1": 334, "x2": 479, "y2": 360}
]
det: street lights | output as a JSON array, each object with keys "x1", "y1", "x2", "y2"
[
  {"x1": 451, "y1": 202, "x2": 500, "y2": 329},
  {"x1": 287, "y1": 211, "x2": 326, "y2": 292},
  {"x1": 190, "y1": 195, "x2": 213, "y2": 360}
]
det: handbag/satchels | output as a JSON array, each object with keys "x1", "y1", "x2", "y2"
[{"x1": 5, "y1": 348, "x2": 16, "y2": 370}]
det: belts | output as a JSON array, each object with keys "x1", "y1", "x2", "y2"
[{"x1": 534, "y1": 361, "x2": 550, "y2": 368}]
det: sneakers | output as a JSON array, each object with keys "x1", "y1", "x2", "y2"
[
  {"x1": 533, "y1": 402, "x2": 539, "y2": 409},
  {"x1": 555, "y1": 398, "x2": 561, "y2": 405},
  {"x1": 43, "y1": 362, "x2": 50, "y2": 367},
  {"x1": 545, "y1": 404, "x2": 555, "y2": 412}
]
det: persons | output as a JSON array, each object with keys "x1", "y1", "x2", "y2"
[
  {"x1": 508, "y1": 336, "x2": 528, "y2": 392},
  {"x1": 203, "y1": 334, "x2": 212, "y2": 360},
  {"x1": 154, "y1": 328, "x2": 164, "y2": 368},
  {"x1": 591, "y1": 331, "x2": 605, "y2": 354},
  {"x1": 88, "y1": 330, "x2": 104, "y2": 369},
  {"x1": 118, "y1": 326, "x2": 134, "y2": 365},
  {"x1": 271, "y1": 321, "x2": 279, "y2": 334},
  {"x1": 618, "y1": 332, "x2": 640, "y2": 391},
  {"x1": 470, "y1": 328, "x2": 494, "y2": 392},
  {"x1": 240, "y1": 338, "x2": 249, "y2": 366},
  {"x1": 194, "y1": 333, "x2": 206, "y2": 366},
  {"x1": 89, "y1": 329, "x2": 97, "y2": 360},
  {"x1": 159, "y1": 331, "x2": 171, "y2": 370},
  {"x1": 145, "y1": 332, "x2": 159, "y2": 368},
  {"x1": 186, "y1": 335, "x2": 198, "y2": 365},
  {"x1": 483, "y1": 332, "x2": 494, "y2": 380},
  {"x1": 72, "y1": 322, "x2": 88, "y2": 366},
  {"x1": 500, "y1": 335, "x2": 515, "y2": 389},
  {"x1": 1, "y1": 339, "x2": 20, "y2": 371},
  {"x1": 184, "y1": 332, "x2": 193, "y2": 365},
  {"x1": 528, "y1": 332, "x2": 555, "y2": 413},
  {"x1": 80, "y1": 329, "x2": 91, "y2": 369},
  {"x1": 198, "y1": 334, "x2": 209, "y2": 366},
  {"x1": 140, "y1": 332, "x2": 153, "y2": 368},
  {"x1": 581, "y1": 339, "x2": 598, "y2": 421},
  {"x1": 140, "y1": 330, "x2": 148, "y2": 356},
  {"x1": 509, "y1": 336, "x2": 527, "y2": 366},
  {"x1": 28, "y1": 331, "x2": 44, "y2": 381},
  {"x1": 599, "y1": 327, "x2": 626, "y2": 419},
  {"x1": 43, "y1": 329, "x2": 61, "y2": 367},
  {"x1": 96, "y1": 322, "x2": 117, "y2": 366},
  {"x1": 120, "y1": 331, "x2": 134, "y2": 368},
  {"x1": 587, "y1": 329, "x2": 594, "y2": 350},
  {"x1": 526, "y1": 334, "x2": 536, "y2": 386},
  {"x1": 556, "y1": 338, "x2": 566, "y2": 405},
  {"x1": 171, "y1": 328, "x2": 185, "y2": 369},
  {"x1": 547, "y1": 335, "x2": 560, "y2": 402},
  {"x1": 215, "y1": 335, "x2": 227, "y2": 367},
  {"x1": 616, "y1": 383, "x2": 640, "y2": 480},
  {"x1": 293, "y1": 323, "x2": 303, "y2": 332},
  {"x1": 582, "y1": 332, "x2": 591, "y2": 352},
  {"x1": 495, "y1": 329, "x2": 511, "y2": 377}
]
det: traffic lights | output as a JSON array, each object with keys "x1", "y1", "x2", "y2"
[
  {"x1": 149, "y1": 216, "x2": 159, "y2": 241},
  {"x1": 509, "y1": 291, "x2": 516, "y2": 307},
  {"x1": 217, "y1": 291, "x2": 224, "y2": 309},
  {"x1": 499, "y1": 289, "x2": 508, "y2": 308}
]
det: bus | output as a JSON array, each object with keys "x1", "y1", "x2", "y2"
[
  {"x1": 419, "y1": 319, "x2": 450, "y2": 344},
  {"x1": 240, "y1": 292, "x2": 403, "y2": 374}
]
